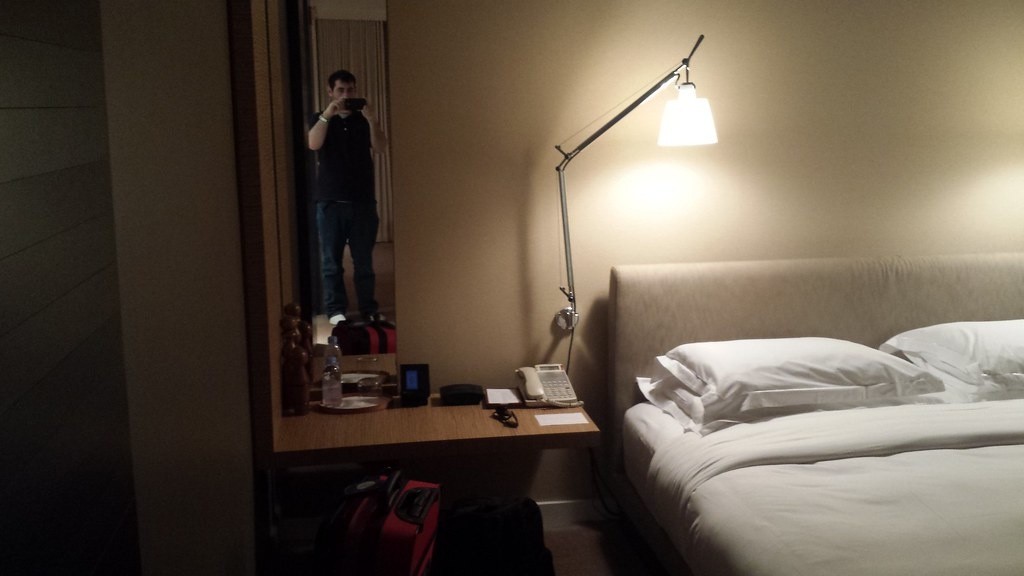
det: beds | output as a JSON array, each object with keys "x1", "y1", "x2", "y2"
[{"x1": 609, "y1": 252, "x2": 1024, "y2": 576}]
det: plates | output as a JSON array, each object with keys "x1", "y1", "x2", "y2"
[{"x1": 320, "y1": 397, "x2": 378, "y2": 409}]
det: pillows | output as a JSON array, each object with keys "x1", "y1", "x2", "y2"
[{"x1": 637, "y1": 319, "x2": 1024, "y2": 438}]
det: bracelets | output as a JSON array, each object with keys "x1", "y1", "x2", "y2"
[{"x1": 319, "y1": 114, "x2": 330, "y2": 124}]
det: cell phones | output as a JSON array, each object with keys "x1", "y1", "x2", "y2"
[{"x1": 343, "y1": 98, "x2": 366, "y2": 110}]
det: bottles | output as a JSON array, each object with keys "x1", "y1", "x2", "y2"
[
  {"x1": 323, "y1": 336, "x2": 341, "y2": 367},
  {"x1": 322, "y1": 357, "x2": 342, "y2": 404}
]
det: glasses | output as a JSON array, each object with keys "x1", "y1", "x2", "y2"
[{"x1": 493, "y1": 406, "x2": 518, "y2": 428}]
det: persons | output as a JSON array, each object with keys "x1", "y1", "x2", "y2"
[{"x1": 309, "y1": 70, "x2": 387, "y2": 328}]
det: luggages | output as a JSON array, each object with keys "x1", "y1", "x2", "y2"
[
  {"x1": 331, "y1": 464, "x2": 442, "y2": 576},
  {"x1": 332, "y1": 313, "x2": 396, "y2": 356},
  {"x1": 430, "y1": 495, "x2": 555, "y2": 576}
]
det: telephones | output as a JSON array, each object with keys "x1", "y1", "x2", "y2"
[{"x1": 514, "y1": 364, "x2": 578, "y2": 408}]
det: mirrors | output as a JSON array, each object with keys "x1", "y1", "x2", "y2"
[{"x1": 286, "y1": 1, "x2": 403, "y2": 397}]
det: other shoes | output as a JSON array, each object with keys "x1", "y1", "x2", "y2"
[
  {"x1": 329, "y1": 314, "x2": 350, "y2": 323},
  {"x1": 363, "y1": 312, "x2": 386, "y2": 321}
]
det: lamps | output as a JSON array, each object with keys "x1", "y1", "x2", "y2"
[{"x1": 553, "y1": 35, "x2": 720, "y2": 331}]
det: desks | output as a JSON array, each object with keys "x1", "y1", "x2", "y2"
[{"x1": 276, "y1": 389, "x2": 600, "y2": 466}]
